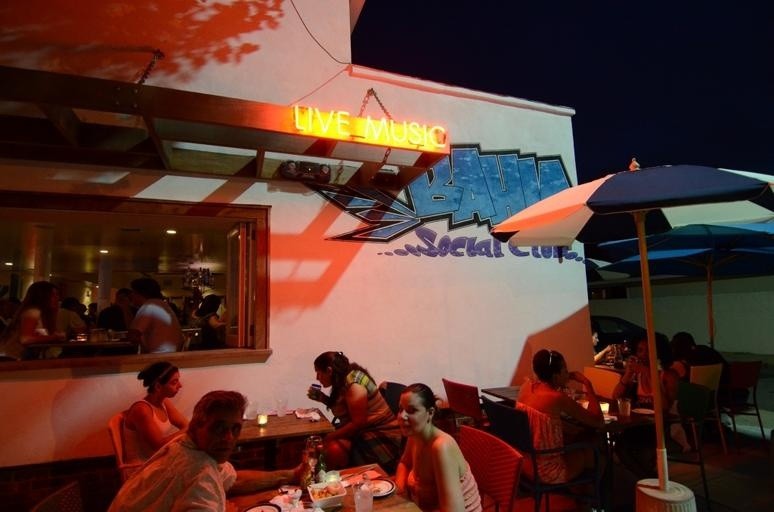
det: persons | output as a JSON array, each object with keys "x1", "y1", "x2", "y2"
[
  {"x1": 305, "y1": 352, "x2": 402, "y2": 469},
  {"x1": 108, "y1": 391, "x2": 308, "y2": 511},
  {"x1": 664, "y1": 344, "x2": 699, "y2": 455},
  {"x1": 397, "y1": 382, "x2": 484, "y2": 510},
  {"x1": 672, "y1": 330, "x2": 730, "y2": 406},
  {"x1": 121, "y1": 361, "x2": 189, "y2": 473},
  {"x1": 3, "y1": 273, "x2": 225, "y2": 359},
  {"x1": 516, "y1": 349, "x2": 616, "y2": 511},
  {"x1": 592, "y1": 332, "x2": 612, "y2": 364},
  {"x1": 615, "y1": 341, "x2": 677, "y2": 478}
]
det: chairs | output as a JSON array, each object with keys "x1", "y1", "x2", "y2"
[
  {"x1": 455, "y1": 426, "x2": 526, "y2": 512},
  {"x1": 689, "y1": 364, "x2": 729, "y2": 461},
  {"x1": 29, "y1": 476, "x2": 106, "y2": 511},
  {"x1": 443, "y1": 381, "x2": 483, "y2": 426},
  {"x1": 584, "y1": 366, "x2": 621, "y2": 401},
  {"x1": 178, "y1": 336, "x2": 193, "y2": 353},
  {"x1": 481, "y1": 403, "x2": 609, "y2": 512},
  {"x1": 108, "y1": 413, "x2": 149, "y2": 480},
  {"x1": 721, "y1": 357, "x2": 765, "y2": 450},
  {"x1": 379, "y1": 382, "x2": 409, "y2": 413}
]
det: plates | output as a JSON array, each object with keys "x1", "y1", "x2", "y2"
[
  {"x1": 632, "y1": 408, "x2": 655, "y2": 415},
  {"x1": 244, "y1": 503, "x2": 282, "y2": 512},
  {"x1": 308, "y1": 481, "x2": 346, "y2": 508},
  {"x1": 360, "y1": 479, "x2": 395, "y2": 498}
]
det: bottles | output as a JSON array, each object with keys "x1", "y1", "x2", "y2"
[
  {"x1": 607, "y1": 340, "x2": 630, "y2": 368},
  {"x1": 299, "y1": 435, "x2": 327, "y2": 494}
]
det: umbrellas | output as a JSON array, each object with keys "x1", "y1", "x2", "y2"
[
  {"x1": 488, "y1": 157, "x2": 774, "y2": 490},
  {"x1": 597, "y1": 224, "x2": 773, "y2": 353}
]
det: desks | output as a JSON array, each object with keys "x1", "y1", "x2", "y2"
[
  {"x1": 482, "y1": 384, "x2": 690, "y2": 512},
  {"x1": 225, "y1": 464, "x2": 423, "y2": 511},
  {"x1": 235, "y1": 409, "x2": 337, "y2": 466},
  {"x1": 25, "y1": 335, "x2": 137, "y2": 359}
]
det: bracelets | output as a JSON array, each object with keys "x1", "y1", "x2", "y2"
[{"x1": 619, "y1": 378, "x2": 629, "y2": 391}]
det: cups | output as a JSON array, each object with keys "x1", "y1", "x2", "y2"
[
  {"x1": 277, "y1": 400, "x2": 287, "y2": 417},
  {"x1": 618, "y1": 399, "x2": 631, "y2": 417},
  {"x1": 576, "y1": 400, "x2": 589, "y2": 409},
  {"x1": 310, "y1": 384, "x2": 321, "y2": 401},
  {"x1": 600, "y1": 402, "x2": 610, "y2": 413},
  {"x1": 256, "y1": 410, "x2": 268, "y2": 427},
  {"x1": 355, "y1": 484, "x2": 373, "y2": 512},
  {"x1": 247, "y1": 402, "x2": 258, "y2": 420}
]
